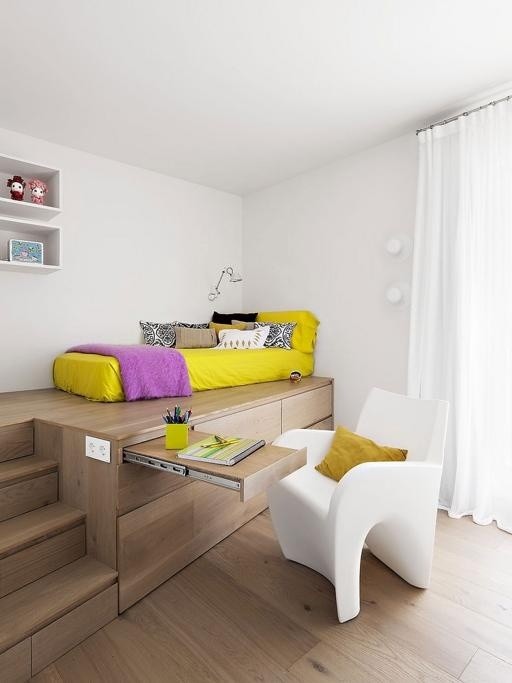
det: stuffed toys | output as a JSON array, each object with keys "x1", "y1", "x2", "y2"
[
  {"x1": 29, "y1": 179, "x2": 48, "y2": 206},
  {"x1": 6, "y1": 175, "x2": 27, "y2": 202}
]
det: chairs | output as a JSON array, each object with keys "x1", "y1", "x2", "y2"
[{"x1": 268, "y1": 382, "x2": 451, "y2": 626}]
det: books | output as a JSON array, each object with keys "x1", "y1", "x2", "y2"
[{"x1": 177, "y1": 432, "x2": 265, "y2": 467}]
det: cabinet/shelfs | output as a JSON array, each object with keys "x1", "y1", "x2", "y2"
[
  {"x1": 0, "y1": 154, "x2": 63, "y2": 272},
  {"x1": 114, "y1": 377, "x2": 335, "y2": 618}
]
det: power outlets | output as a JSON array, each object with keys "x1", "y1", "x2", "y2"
[{"x1": 85, "y1": 435, "x2": 112, "y2": 465}]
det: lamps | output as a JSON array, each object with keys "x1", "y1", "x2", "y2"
[{"x1": 207, "y1": 266, "x2": 244, "y2": 300}]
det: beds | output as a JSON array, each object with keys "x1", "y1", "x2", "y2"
[{"x1": 51, "y1": 308, "x2": 320, "y2": 404}]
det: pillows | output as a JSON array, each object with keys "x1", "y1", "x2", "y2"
[
  {"x1": 314, "y1": 423, "x2": 410, "y2": 485},
  {"x1": 138, "y1": 310, "x2": 298, "y2": 351}
]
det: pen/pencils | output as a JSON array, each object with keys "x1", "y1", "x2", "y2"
[
  {"x1": 162, "y1": 403, "x2": 192, "y2": 424},
  {"x1": 201, "y1": 435, "x2": 238, "y2": 448}
]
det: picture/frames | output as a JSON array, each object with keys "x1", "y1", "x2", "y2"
[{"x1": 8, "y1": 238, "x2": 45, "y2": 265}]
